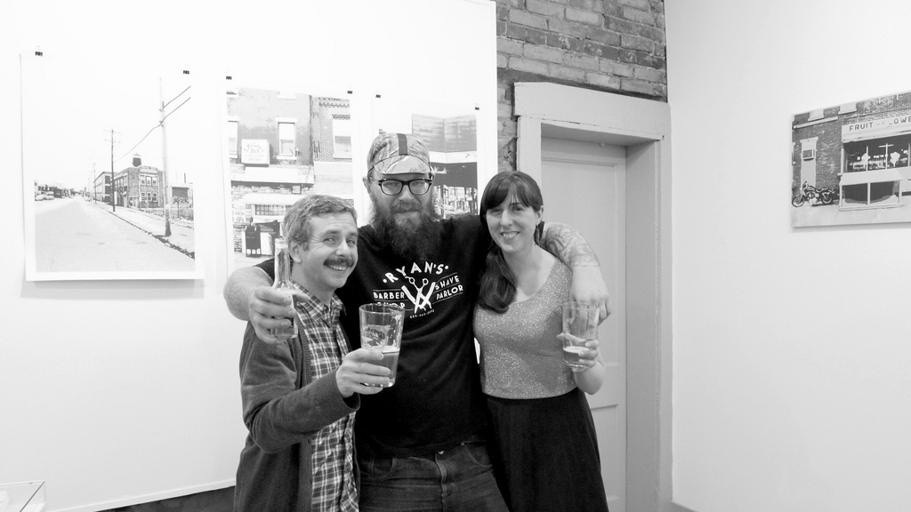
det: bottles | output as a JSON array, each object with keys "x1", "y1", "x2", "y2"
[{"x1": 269, "y1": 237, "x2": 300, "y2": 338}]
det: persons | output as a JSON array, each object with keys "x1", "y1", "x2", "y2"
[
  {"x1": 471, "y1": 170, "x2": 609, "y2": 511},
  {"x1": 222, "y1": 130, "x2": 613, "y2": 512},
  {"x1": 231, "y1": 194, "x2": 393, "y2": 511}
]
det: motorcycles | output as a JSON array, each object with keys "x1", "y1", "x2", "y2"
[{"x1": 792, "y1": 180, "x2": 835, "y2": 206}]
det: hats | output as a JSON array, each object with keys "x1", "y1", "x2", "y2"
[{"x1": 364, "y1": 131, "x2": 432, "y2": 176}]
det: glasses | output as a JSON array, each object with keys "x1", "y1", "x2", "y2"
[{"x1": 366, "y1": 175, "x2": 434, "y2": 197}]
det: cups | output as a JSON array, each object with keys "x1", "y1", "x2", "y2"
[
  {"x1": 561, "y1": 301, "x2": 601, "y2": 369},
  {"x1": 358, "y1": 302, "x2": 405, "y2": 390}
]
